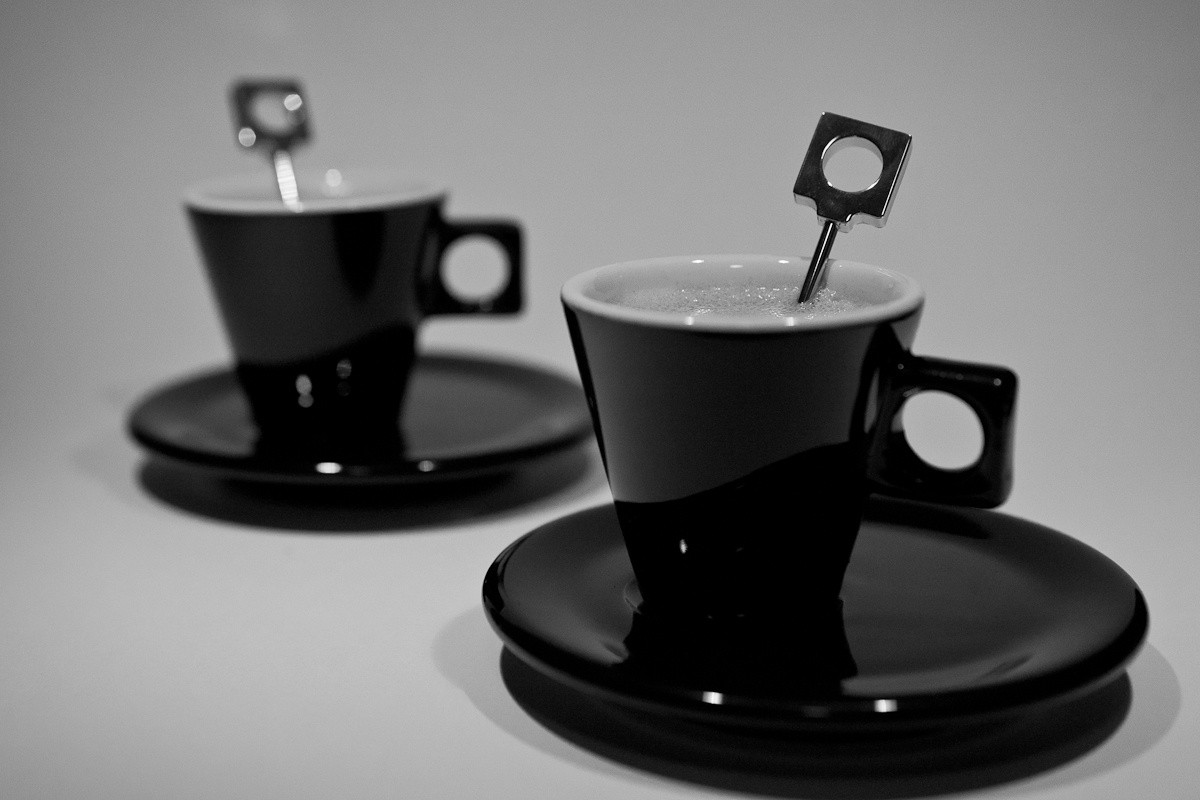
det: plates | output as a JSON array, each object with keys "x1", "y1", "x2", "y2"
[
  {"x1": 479, "y1": 500, "x2": 1149, "y2": 731},
  {"x1": 129, "y1": 355, "x2": 594, "y2": 488}
]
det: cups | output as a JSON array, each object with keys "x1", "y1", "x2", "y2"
[
  {"x1": 186, "y1": 169, "x2": 529, "y2": 444},
  {"x1": 562, "y1": 256, "x2": 1018, "y2": 613}
]
